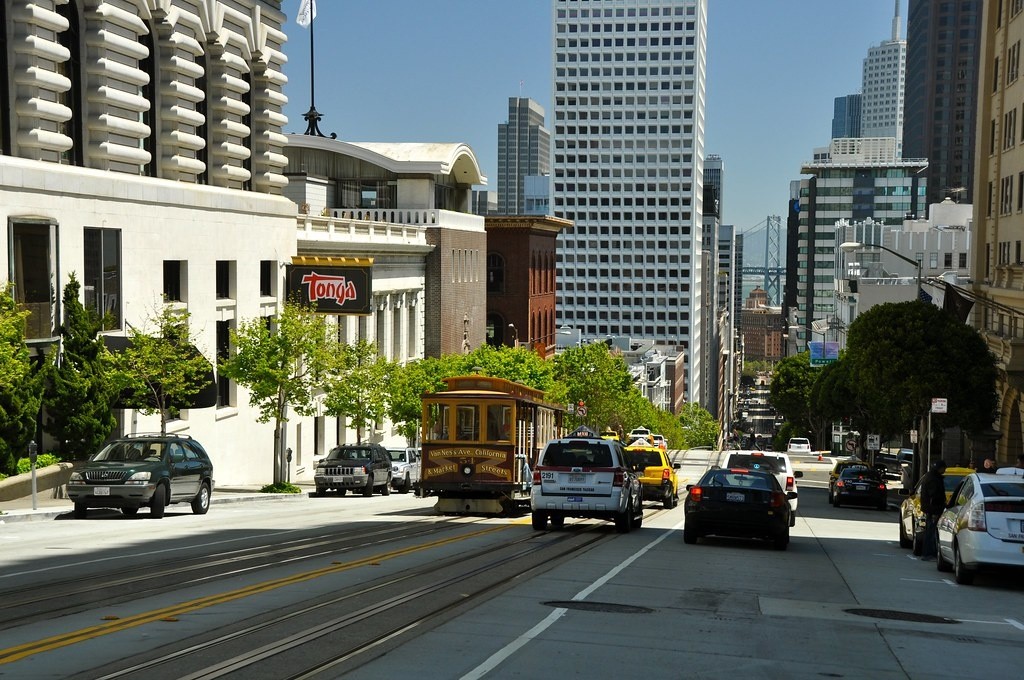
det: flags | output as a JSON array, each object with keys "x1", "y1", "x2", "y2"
[{"x1": 296, "y1": 0, "x2": 317, "y2": 28}]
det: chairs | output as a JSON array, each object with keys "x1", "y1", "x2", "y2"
[
  {"x1": 713, "y1": 476, "x2": 729, "y2": 485},
  {"x1": 159, "y1": 447, "x2": 175, "y2": 464},
  {"x1": 751, "y1": 479, "x2": 767, "y2": 488},
  {"x1": 143, "y1": 449, "x2": 157, "y2": 461},
  {"x1": 124, "y1": 447, "x2": 141, "y2": 461},
  {"x1": 399, "y1": 454, "x2": 404, "y2": 462}
]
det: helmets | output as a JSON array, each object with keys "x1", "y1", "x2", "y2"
[{"x1": 931, "y1": 460, "x2": 944, "y2": 472}]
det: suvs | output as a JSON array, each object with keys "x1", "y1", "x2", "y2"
[
  {"x1": 315, "y1": 442, "x2": 393, "y2": 497},
  {"x1": 65, "y1": 430, "x2": 215, "y2": 519},
  {"x1": 531, "y1": 436, "x2": 643, "y2": 534},
  {"x1": 385, "y1": 446, "x2": 421, "y2": 493}
]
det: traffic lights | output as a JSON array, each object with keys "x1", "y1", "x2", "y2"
[{"x1": 578, "y1": 397, "x2": 586, "y2": 407}]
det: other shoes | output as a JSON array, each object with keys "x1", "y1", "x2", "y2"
[{"x1": 921, "y1": 554, "x2": 937, "y2": 562}]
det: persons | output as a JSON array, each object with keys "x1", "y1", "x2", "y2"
[
  {"x1": 1016, "y1": 457, "x2": 1023, "y2": 467},
  {"x1": 956, "y1": 462, "x2": 977, "y2": 471},
  {"x1": 921, "y1": 462, "x2": 947, "y2": 562},
  {"x1": 487, "y1": 411, "x2": 500, "y2": 440},
  {"x1": 456, "y1": 423, "x2": 470, "y2": 440},
  {"x1": 434, "y1": 423, "x2": 448, "y2": 439}
]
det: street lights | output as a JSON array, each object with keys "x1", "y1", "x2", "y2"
[
  {"x1": 840, "y1": 240, "x2": 921, "y2": 492},
  {"x1": 789, "y1": 326, "x2": 827, "y2": 451}
]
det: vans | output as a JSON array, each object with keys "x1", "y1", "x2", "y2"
[
  {"x1": 717, "y1": 450, "x2": 798, "y2": 527},
  {"x1": 787, "y1": 436, "x2": 813, "y2": 453}
]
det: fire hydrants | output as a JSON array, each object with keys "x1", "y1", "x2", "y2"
[{"x1": 817, "y1": 450, "x2": 823, "y2": 461}]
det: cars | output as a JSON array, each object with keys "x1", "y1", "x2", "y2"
[
  {"x1": 897, "y1": 467, "x2": 975, "y2": 554},
  {"x1": 621, "y1": 446, "x2": 680, "y2": 508},
  {"x1": 827, "y1": 460, "x2": 875, "y2": 504},
  {"x1": 831, "y1": 467, "x2": 889, "y2": 512},
  {"x1": 935, "y1": 471, "x2": 1024, "y2": 587},
  {"x1": 599, "y1": 424, "x2": 668, "y2": 450},
  {"x1": 683, "y1": 467, "x2": 798, "y2": 550}
]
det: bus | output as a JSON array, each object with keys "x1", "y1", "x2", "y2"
[{"x1": 419, "y1": 376, "x2": 568, "y2": 516}]
